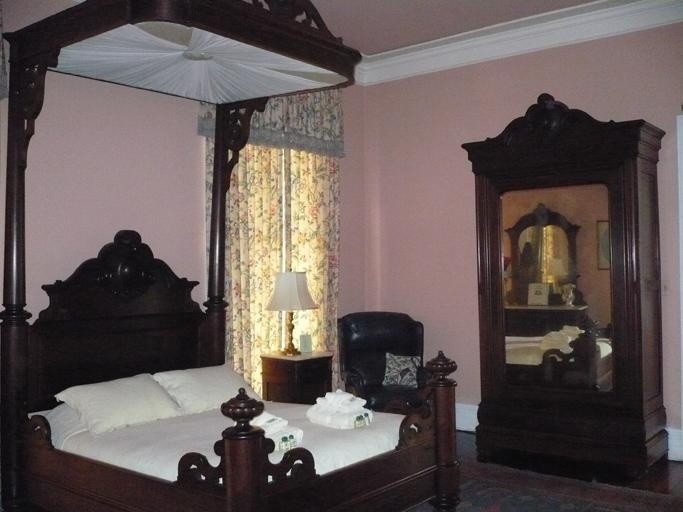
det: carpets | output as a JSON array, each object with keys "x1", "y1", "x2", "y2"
[{"x1": 409, "y1": 451, "x2": 682, "y2": 510}]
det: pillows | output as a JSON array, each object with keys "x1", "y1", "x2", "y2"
[
  {"x1": 53, "y1": 364, "x2": 262, "y2": 435},
  {"x1": 381, "y1": 351, "x2": 422, "y2": 389}
]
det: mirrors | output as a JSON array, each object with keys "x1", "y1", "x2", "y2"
[
  {"x1": 502, "y1": 201, "x2": 588, "y2": 307},
  {"x1": 460, "y1": 92, "x2": 669, "y2": 480}
]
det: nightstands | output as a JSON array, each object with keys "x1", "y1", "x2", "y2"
[{"x1": 260, "y1": 350, "x2": 334, "y2": 405}]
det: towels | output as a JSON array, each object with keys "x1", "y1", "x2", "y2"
[
  {"x1": 539, "y1": 325, "x2": 586, "y2": 353},
  {"x1": 234, "y1": 411, "x2": 303, "y2": 451},
  {"x1": 306, "y1": 390, "x2": 373, "y2": 430}
]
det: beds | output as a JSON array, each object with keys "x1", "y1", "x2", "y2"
[
  {"x1": 2, "y1": 229, "x2": 461, "y2": 512},
  {"x1": 506, "y1": 322, "x2": 613, "y2": 399}
]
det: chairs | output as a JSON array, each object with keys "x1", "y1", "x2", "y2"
[{"x1": 336, "y1": 311, "x2": 433, "y2": 411}]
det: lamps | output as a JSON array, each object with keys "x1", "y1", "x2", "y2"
[{"x1": 264, "y1": 268, "x2": 319, "y2": 357}]
